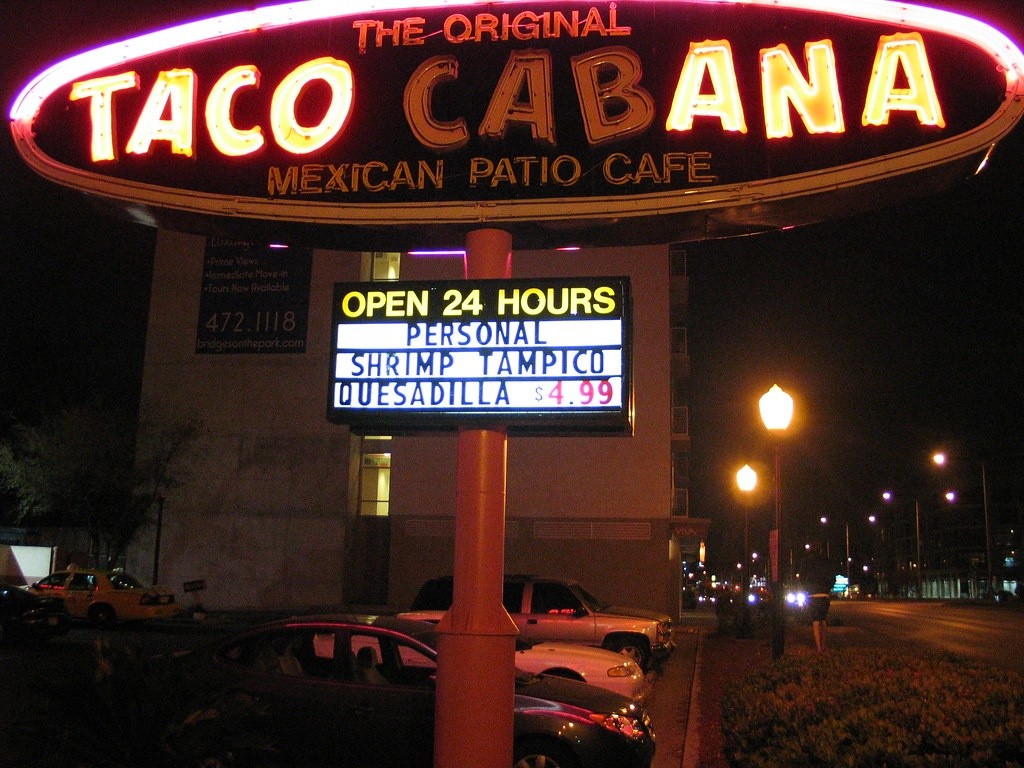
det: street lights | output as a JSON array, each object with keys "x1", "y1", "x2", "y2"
[
  {"x1": 757, "y1": 385, "x2": 794, "y2": 654},
  {"x1": 879, "y1": 492, "x2": 923, "y2": 601},
  {"x1": 930, "y1": 451, "x2": 995, "y2": 608},
  {"x1": 819, "y1": 518, "x2": 851, "y2": 602},
  {"x1": 733, "y1": 464, "x2": 758, "y2": 636}
]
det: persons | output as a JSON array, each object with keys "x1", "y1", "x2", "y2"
[{"x1": 798, "y1": 540, "x2": 837, "y2": 656}]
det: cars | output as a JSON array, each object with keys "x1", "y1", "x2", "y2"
[
  {"x1": 394, "y1": 612, "x2": 648, "y2": 701},
  {"x1": 0, "y1": 579, "x2": 75, "y2": 645},
  {"x1": 16, "y1": 569, "x2": 179, "y2": 628},
  {"x1": 132, "y1": 616, "x2": 656, "y2": 767}
]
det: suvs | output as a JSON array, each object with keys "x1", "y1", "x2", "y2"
[{"x1": 411, "y1": 574, "x2": 676, "y2": 670}]
